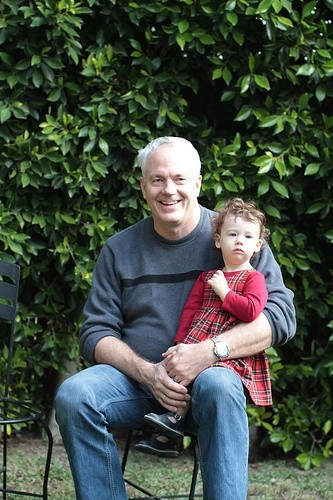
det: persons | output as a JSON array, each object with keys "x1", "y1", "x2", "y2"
[
  {"x1": 133, "y1": 198, "x2": 273, "y2": 459},
  {"x1": 54, "y1": 136, "x2": 297, "y2": 500}
]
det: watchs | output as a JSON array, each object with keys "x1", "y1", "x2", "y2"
[{"x1": 209, "y1": 336, "x2": 231, "y2": 363}]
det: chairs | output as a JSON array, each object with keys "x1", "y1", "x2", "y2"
[
  {"x1": 0, "y1": 260, "x2": 53, "y2": 500},
  {"x1": 121, "y1": 428, "x2": 200, "y2": 500}
]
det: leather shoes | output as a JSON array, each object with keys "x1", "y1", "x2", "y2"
[
  {"x1": 144, "y1": 412, "x2": 186, "y2": 440},
  {"x1": 135, "y1": 433, "x2": 179, "y2": 458}
]
